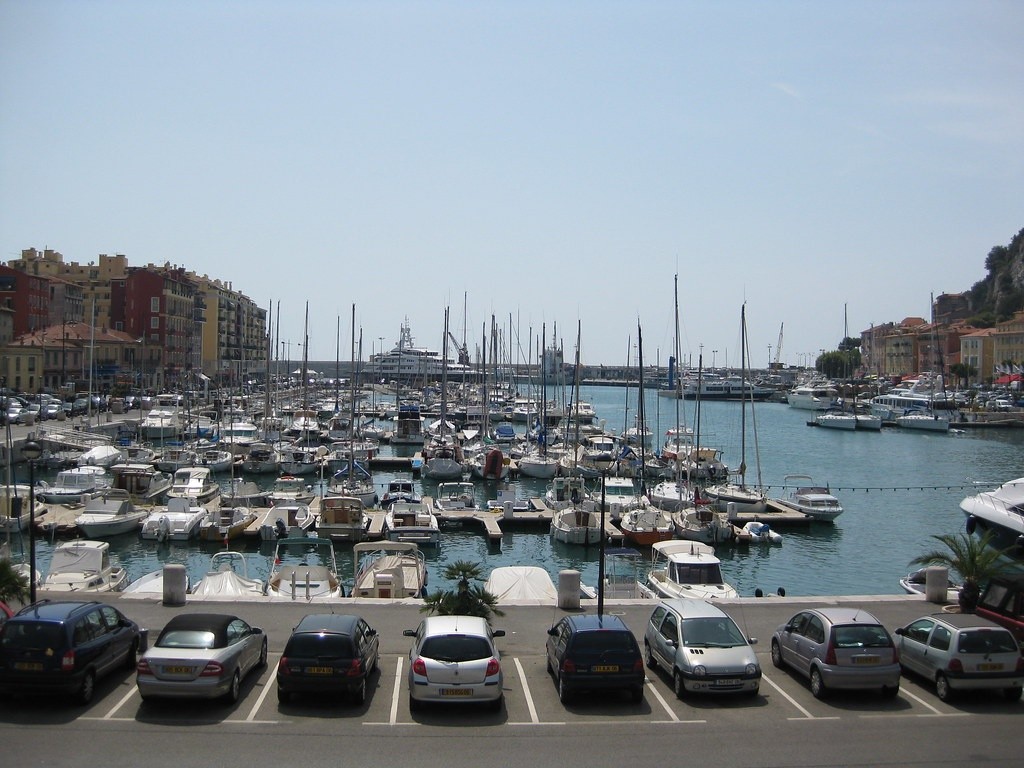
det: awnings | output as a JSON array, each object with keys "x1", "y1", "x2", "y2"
[{"x1": 994, "y1": 374, "x2": 1024, "y2": 383}]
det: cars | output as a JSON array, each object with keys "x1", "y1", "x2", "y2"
[
  {"x1": 403, "y1": 615, "x2": 505, "y2": 714},
  {"x1": 136, "y1": 614, "x2": 267, "y2": 705},
  {"x1": 0, "y1": 378, "x2": 350, "y2": 427},
  {"x1": 277, "y1": 612, "x2": 379, "y2": 705}
]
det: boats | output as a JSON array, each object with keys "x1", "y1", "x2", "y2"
[
  {"x1": 776, "y1": 475, "x2": 843, "y2": 521},
  {"x1": 960, "y1": 477, "x2": 1024, "y2": 565},
  {"x1": 35, "y1": 541, "x2": 134, "y2": 592},
  {"x1": 191, "y1": 552, "x2": 265, "y2": 596},
  {"x1": 484, "y1": 567, "x2": 559, "y2": 602},
  {"x1": 646, "y1": 540, "x2": 740, "y2": 599},
  {"x1": 121, "y1": 569, "x2": 189, "y2": 593},
  {"x1": 579, "y1": 548, "x2": 659, "y2": 599},
  {"x1": 264, "y1": 538, "x2": 346, "y2": 599},
  {"x1": 347, "y1": 540, "x2": 426, "y2": 599}
]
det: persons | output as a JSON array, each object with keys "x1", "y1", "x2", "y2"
[{"x1": 754, "y1": 587, "x2": 786, "y2": 597}]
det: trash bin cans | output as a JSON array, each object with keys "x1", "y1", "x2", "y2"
[
  {"x1": 558, "y1": 570, "x2": 581, "y2": 608},
  {"x1": 925, "y1": 567, "x2": 949, "y2": 603},
  {"x1": 163, "y1": 564, "x2": 186, "y2": 606}
]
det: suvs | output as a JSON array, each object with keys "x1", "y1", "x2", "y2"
[
  {"x1": 0, "y1": 599, "x2": 139, "y2": 707},
  {"x1": 891, "y1": 614, "x2": 1024, "y2": 703},
  {"x1": 545, "y1": 614, "x2": 645, "y2": 701},
  {"x1": 771, "y1": 608, "x2": 901, "y2": 702},
  {"x1": 974, "y1": 573, "x2": 1024, "y2": 656}
]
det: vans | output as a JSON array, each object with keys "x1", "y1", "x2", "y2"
[{"x1": 644, "y1": 599, "x2": 762, "y2": 700}]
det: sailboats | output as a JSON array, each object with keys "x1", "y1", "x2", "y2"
[
  {"x1": 0, "y1": 276, "x2": 814, "y2": 545},
  {"x1": 676, "y1": 293, "x2": 1017, "y2": 432}
]
